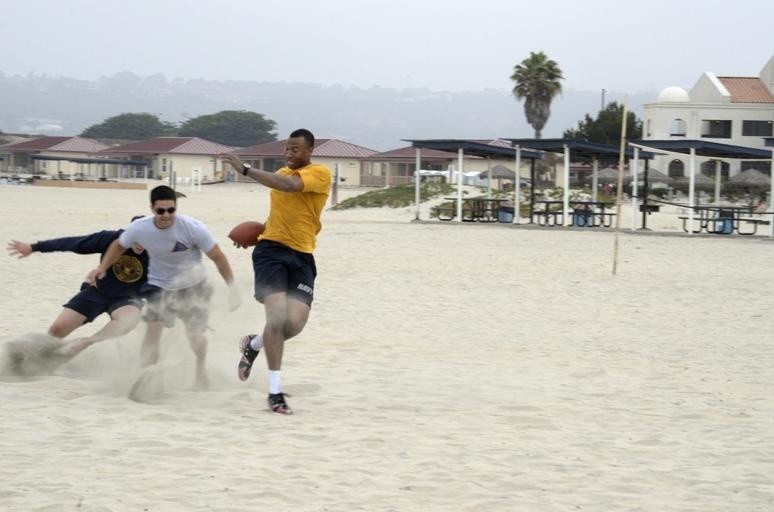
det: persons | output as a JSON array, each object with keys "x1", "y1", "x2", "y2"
[
  {"x1": 5, "y1": 215, "x2": 145, "y2": 362},
  {"x1": 218, "y1": 128, "x2": 331, "y2": 415},
  {"x1": 84, "y1": 186, "x2": 240, "y2": 392}
]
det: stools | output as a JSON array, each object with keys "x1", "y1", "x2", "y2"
[
  {"x1": 431, "y1": 205, "x2": 621, "y2": 227},
  {"x1": 677, "y1": 214, "x2": 771, "y2": 237}
]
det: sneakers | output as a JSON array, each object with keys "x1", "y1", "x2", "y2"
[
  {"x1": 237, "y1": 333, "x2": 261, "y2": 380},
  {"x1": 266, "y1": 391, "x2": 293, "y2": 415}
]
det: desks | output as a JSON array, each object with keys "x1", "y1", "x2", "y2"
[
  {"x1": 443, "y1": 196, "x2": 616, "y2": 228},
  {"x1": 693, "y1": 203, "x2": 758, "y2": 236}
]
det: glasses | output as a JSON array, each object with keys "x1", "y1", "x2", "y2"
[{"x1": 156, "y1": 206, "x2": 175, "y2": 215}]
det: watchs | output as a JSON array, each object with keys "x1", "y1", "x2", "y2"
[{"x1": 242, "y1": 163, "x2": 251, "y2": 176}]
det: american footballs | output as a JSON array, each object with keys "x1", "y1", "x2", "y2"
[{"x1": 228, "y1": 221, "x2": 265, "y2": 246}]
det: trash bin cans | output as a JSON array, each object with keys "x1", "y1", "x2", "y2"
[
  {"x1": 720, "y1": 211, "x2": 733, "y2": 234},
  {"x1": 498, "y1": 206, "x2": 514, "y2": 223}
]
